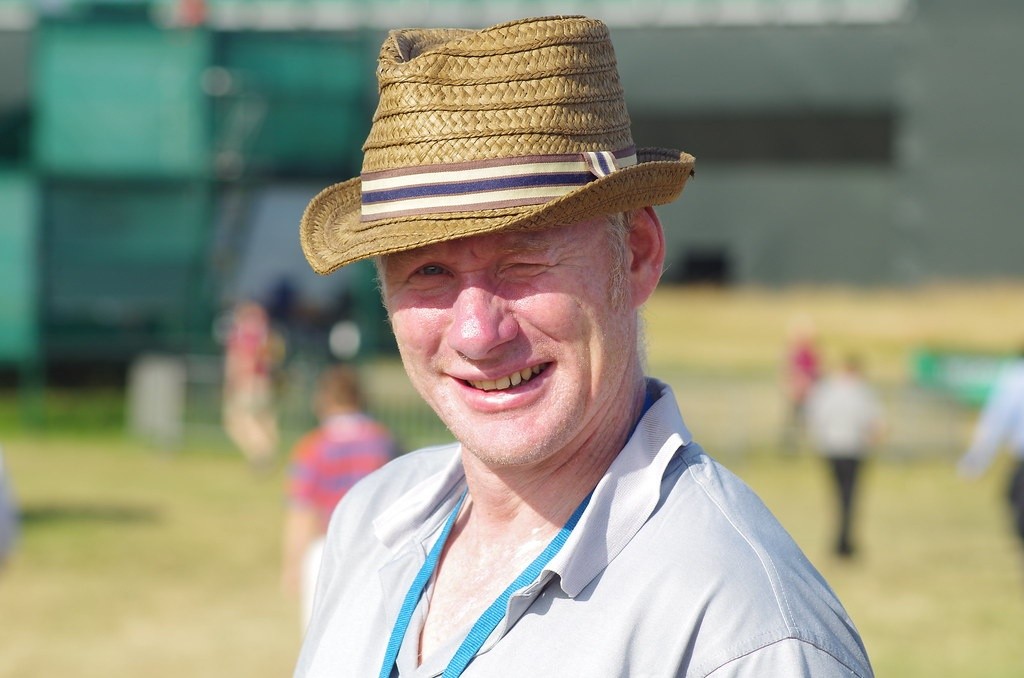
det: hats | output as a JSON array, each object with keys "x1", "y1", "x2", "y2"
[{"x1": 300, "y1": 13, "x2": 696, "y2": 277}]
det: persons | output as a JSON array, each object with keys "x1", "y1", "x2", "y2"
[
  {"x1": 959, "y1": 352, "x2": 1024, "y2": 552},
  {"x1": 802, "y1": 345, "x2": 887, "y2": 554},
  {"x1": 288, "y1": 364, "x2": 404, "y2": 634},
  {"x1": 221, "y1": 295, "x2": 283, "y2": 461},
  {"x1": 296, "y1": 15, "x2": 875, "y2": 678}
]
